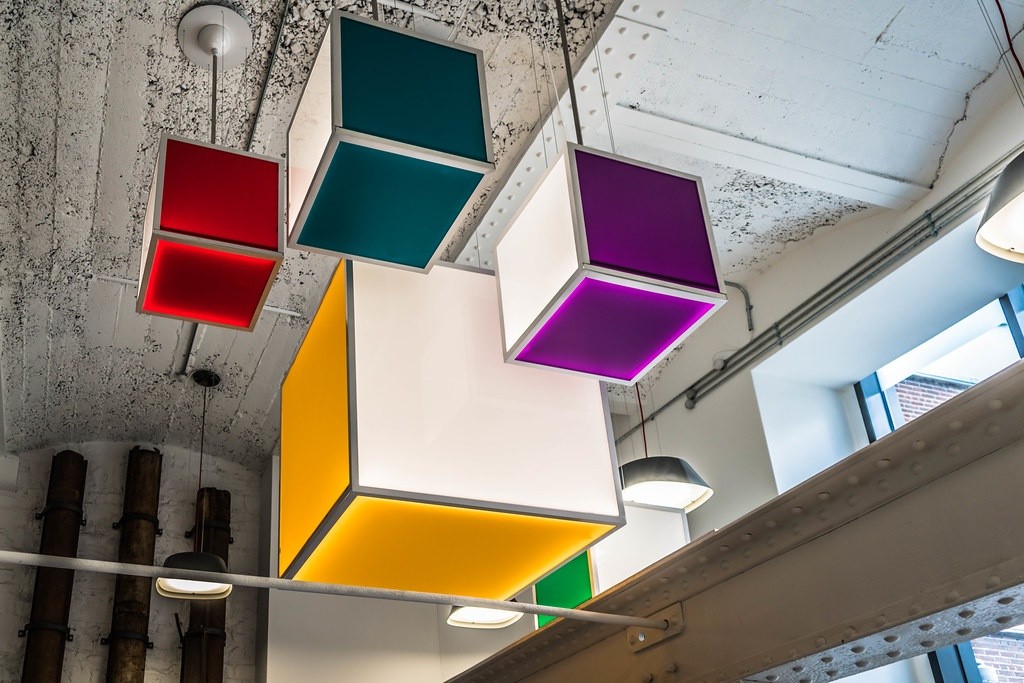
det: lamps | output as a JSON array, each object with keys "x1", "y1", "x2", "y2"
[
  {"x1": 617, "y1": 381, "x2": 714, "y2": 515},
  {"x1": 448, "y1": 596, "x2": 525, "y2": 628},
  {"x1": 154, "y1": 368, "x2": 235, "y2": 600},
  {"x1": 285, "y1": 1, "x2": 497, "y2": 274},
  {"x1": 275, "y1": 256, "x2": 626, "y2": 603},
  {"x1": 136, "y1": 4, "x2": 285, "y2": 333},
  {"x1": 976, "y1": 0, "x2": 1024, "y2": 265},
  {"x1": 495, "y1": 0, "x2": 726, "y2": 387}
]
